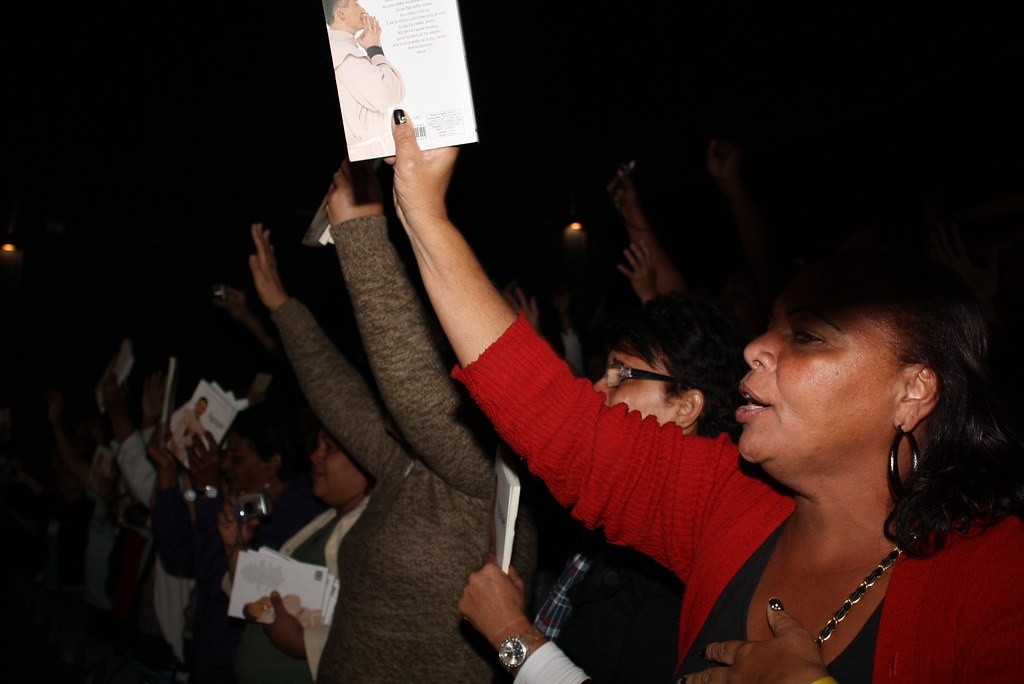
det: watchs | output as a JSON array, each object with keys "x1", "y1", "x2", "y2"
[
  {"x1": 498, "y1": 628, "x2": 546, "y2": 672},
  {"x1": 199, "y1": 486, "x2": 221, "y2": 499},
  {"x1": 185, "y1": 489, "x2": 201, "y2": 502}
]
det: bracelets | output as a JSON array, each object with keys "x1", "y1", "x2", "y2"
[{"x1": 811, "y1": 676, "x2": 838, "y2": 684}]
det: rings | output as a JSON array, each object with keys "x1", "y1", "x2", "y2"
[{"x1": 106, "y1": 387, "x2": 110, "y2": 391}]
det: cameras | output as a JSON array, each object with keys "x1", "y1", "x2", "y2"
[
  {"x1": 236, "y1": 492, "x2": 276, "y2": 529},
  {"x1": 214, "y1": 284, "x2": 225, "y2": 301}
]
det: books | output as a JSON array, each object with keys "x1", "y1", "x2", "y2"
[
  {"x1": 97, "y1": 341, "x2": 133, "y2": 410},
  {"x1": 301, "y1": 152, "x2": 381, "y2": 246},
  {"x1": 323, "y1": 0, "x2": 479, "y2": 162},
  {"x1": 93, "y1": 446, "x2": 117, "y2": 478},
  {"x1": 490, "y1": 442, "x2": 521, "y2": 574},
  {"x1": 167, "y1": 380, "x2": 237, "y2": 470},
  {"x1": 226, "y1": 545, "x2": 339, "y2": 627}
]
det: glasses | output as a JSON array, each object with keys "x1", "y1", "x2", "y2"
[{"x1": 604, "y1": 363, "x2": 695, "y2": 389}]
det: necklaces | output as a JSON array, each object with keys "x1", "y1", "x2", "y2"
[{"x1": 815, "y1": 528, "x2": 920, "y2": 650}]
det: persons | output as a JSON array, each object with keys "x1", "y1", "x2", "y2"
[
  {"x1": 0, "y1": 373, "x2": 373, "y2": 684},
  {"x1": 392, "y1": 109, "x2": 1024, "y2": 684},
  {"x1": 250, "y1": 159, "x2": 537, "y2": 684},
  {"x1": 323, "y1": 0, "x2": 405, "y2": 162}
]
